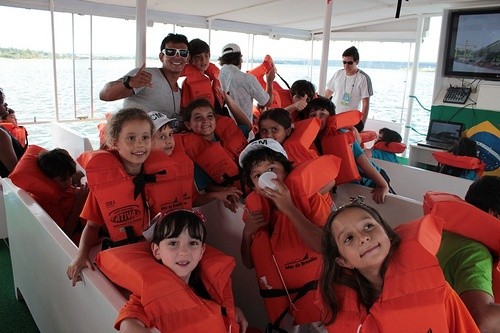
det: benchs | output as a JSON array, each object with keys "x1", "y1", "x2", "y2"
[
  {"x1": 329, "y1": 182, "x2": 425, "y2": 228},
  {"x1": 370, "y1": 157, "x2": 474, "y2": 204},
  {"x1": 191, "y1": 190, "x2": 295, "y2": 333},
  {"x1": 1, "y1": 177, "x2": 161, "y2": 333},
  {"x1": 54, "y1": 122, "x2": 93, "y2": 185}
]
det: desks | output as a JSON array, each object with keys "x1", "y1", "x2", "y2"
[{"x1": 409, "y1": 142, "x2": 447, "y2": 167}]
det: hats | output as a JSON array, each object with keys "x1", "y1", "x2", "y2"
[
  {"x1": 238, "y1": 137, "x2": 288, "y2": 169},
  {"x1": 148, "y1": 111, "x2": 176, "y2": 135},
  {"x1": 219, "y1": 43, "x2": 241, "y2": 58}
]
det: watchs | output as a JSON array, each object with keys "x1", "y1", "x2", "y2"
[{"x1": 123, "y1": 75, "x2": 134, "y2": 89}]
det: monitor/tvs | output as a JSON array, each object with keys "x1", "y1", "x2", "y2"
[{"x1": 443, "y1": 9, "x2": 500, "y2": 81}]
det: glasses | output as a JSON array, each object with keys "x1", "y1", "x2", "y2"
[
  {"x1": 161, "y1": 47, "x2": 190, "y2": 58},
  {"x1": 343, "y1": 60, "x2": 356, "y2": 65}
]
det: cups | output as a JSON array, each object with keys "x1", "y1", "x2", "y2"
[{"x1": 258, "y1": 171, "x2": 278, "y2": 193}]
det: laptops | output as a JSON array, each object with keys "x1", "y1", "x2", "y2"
[{"x1": 417, "y1": 120, "x2": 464, "y2": 151}]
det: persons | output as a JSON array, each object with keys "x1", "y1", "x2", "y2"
[
  {"x1": 239, "y1": 138, "x2": 342, "y2": 333},
  {"x1": 324, "y1": 46, "x2": 373, "y2": 132},
  {"x1": 99, "y1": 33, "x2": 190, "y2": 133},
  {"x1": 95, "y1": 206, "x2": 249, "y2": 333},
  {"x1": 423, "y1": 174, "x2": 500, "y2": 333},
  {"x1": 437, "y1": 137, "x2": 485, "y2": 181},
  {"x1": 312, "y1": 194, "x2": 483, "y2": 333},
  {"x1": 370, "y1": 128, "x2": 407, "y2": 163},
  {"x1": 0, "y1": 39, "x2": 397, "y2": 288}
]
